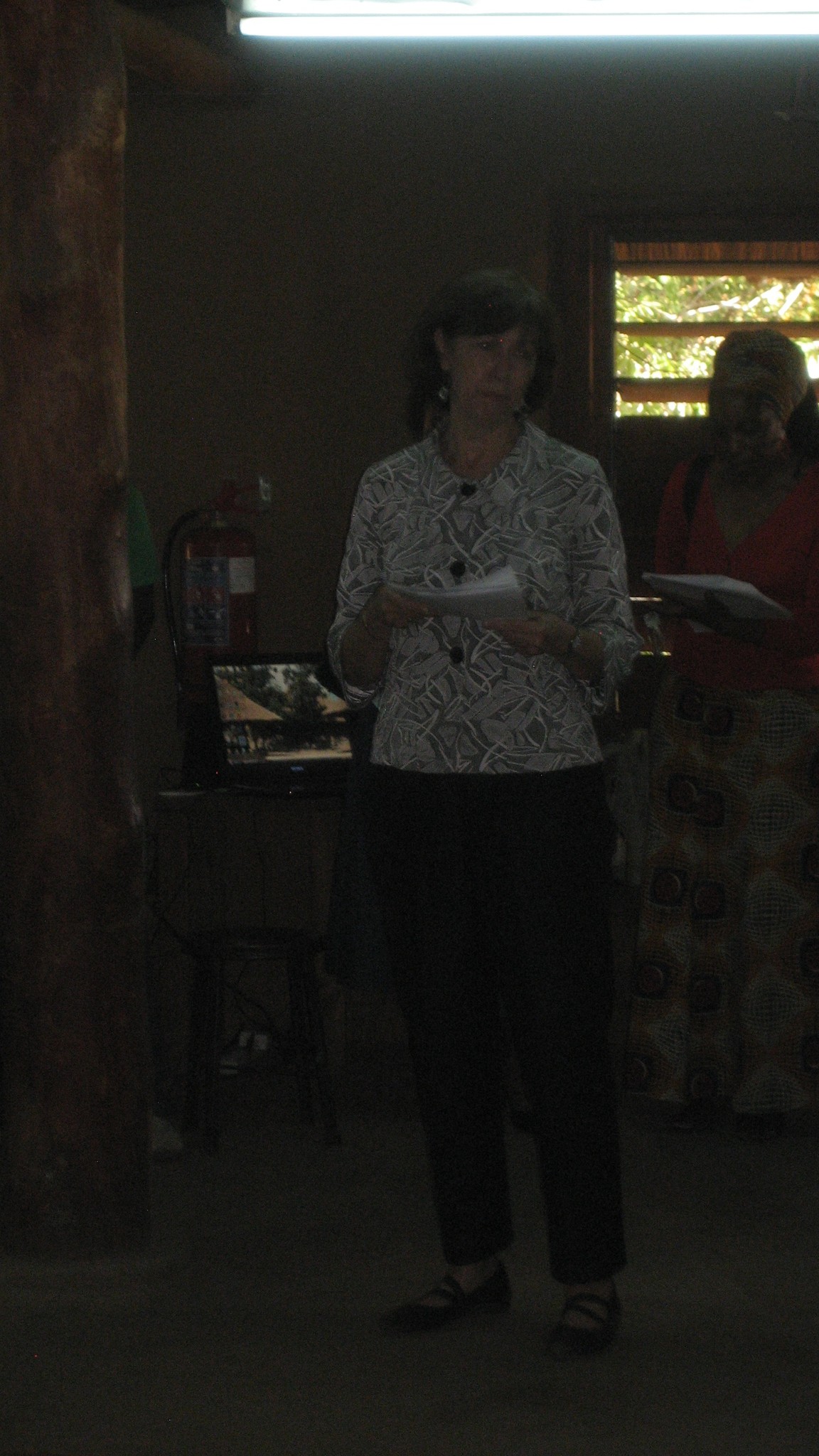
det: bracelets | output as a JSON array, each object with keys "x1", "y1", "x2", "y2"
[
  {"x1": 560, "y1": 625, "x2": 578, "y2": 657},
  {"x1": 360, "y1": 614, "x2": 393, "y2": 643}
]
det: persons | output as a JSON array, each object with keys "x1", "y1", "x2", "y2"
[
  {"x1": 327, "y1": 266, "x2": 641, "y2": 1356},
  {"x1": 626, "y1": 327, "x2": 819, "y2": 1142}
]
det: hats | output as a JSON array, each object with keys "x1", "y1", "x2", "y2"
[{"x1": 707, "y1": 325, "x2": 809, "y2": 425}]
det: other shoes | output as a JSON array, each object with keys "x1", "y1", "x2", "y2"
[
  {"x1": 735, "y1": 1103, "x2": 817, "y2": 1140},
  {"x1": 384, "y1": 1261, "x2": 513, "y2": 1335},
  {"x1": 648, "y1": 1102, "x2": 699, "y2": 1134},
  {"x1": 544, "y1": 1282, "x2": 626, "y2": 1353}
]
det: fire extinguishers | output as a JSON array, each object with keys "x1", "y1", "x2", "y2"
[{"x1": 160, "y1": 481, "x2": 271, "y2": 734}]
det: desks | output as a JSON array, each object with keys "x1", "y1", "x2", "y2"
[{"x1": 154, "y1": 779, "x2": 351, "y2": 1146}]
